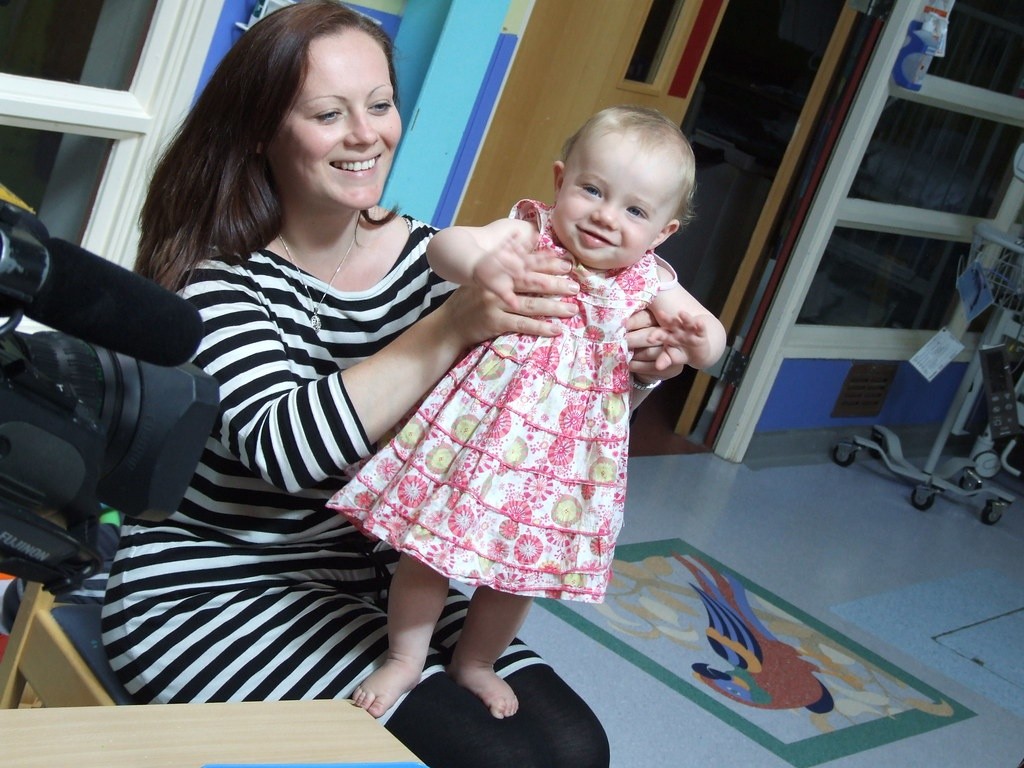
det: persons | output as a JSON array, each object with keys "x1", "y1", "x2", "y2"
[
  {"x1": 100, "y1": 0, "x2": 684, "y2": 768},
  {"x1": 326, "y1": 105, "x2": 727, "y2": 718}
]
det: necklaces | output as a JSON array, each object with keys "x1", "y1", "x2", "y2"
[{"x1": 278, "y1": 233, "x2": 356, "y2": 334}]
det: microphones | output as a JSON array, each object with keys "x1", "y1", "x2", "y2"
[{"x1": 0, "y1": 224, "x2": 204, "y2": 366}]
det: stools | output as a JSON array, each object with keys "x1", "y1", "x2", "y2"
[{"x1": 0, "y1": 579, "x2": 140, "y2": 708}]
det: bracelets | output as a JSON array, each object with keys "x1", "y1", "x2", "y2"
[{"x1": 634, "y1": 380, "x2": 661, "y2": 393}]
